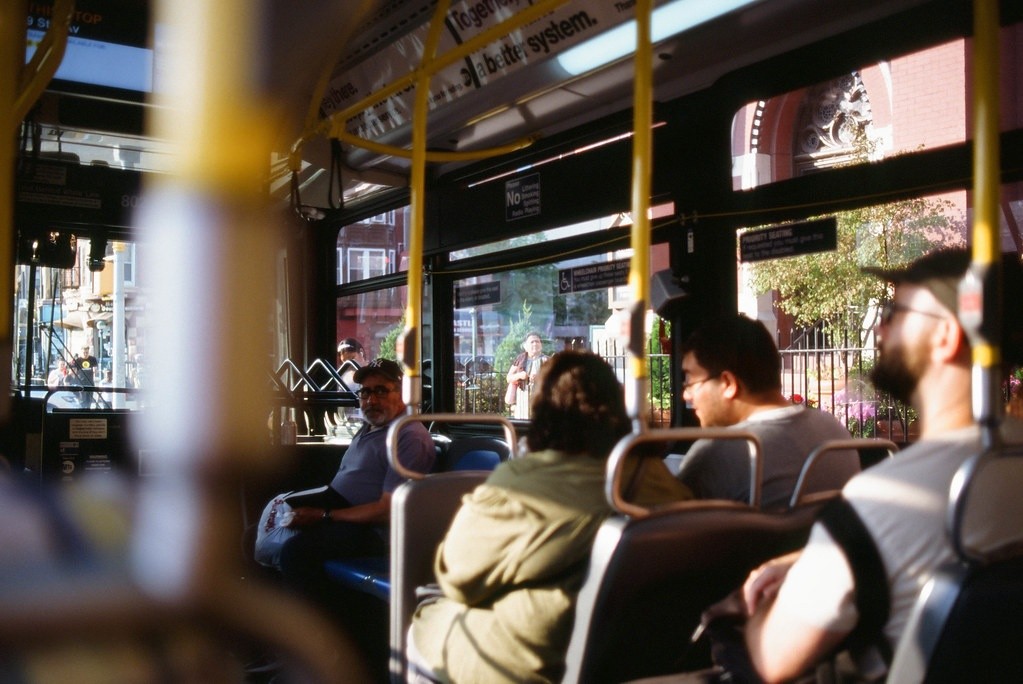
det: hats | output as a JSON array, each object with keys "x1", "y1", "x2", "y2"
[
  {"x1": 352, "y1": 358, "x2": 403, "y2": 385},
  {"x1": 861, "y1": 249, "x2": 1023, "y2": 366}
]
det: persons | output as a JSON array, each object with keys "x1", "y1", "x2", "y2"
[
  {"x1": 255, "y1": 358, "x2": 435, "y2": 596},
  {"x1": 402, "y1": 348, "x2": 701, "y2": 682},
  {"x1": 49, "y1": 345, "x2": 122, "y2": 409},
  {"x1": 674, "y1": 313, "x2": 864, "y2": 553},
  {"x1": 336, "y1": 337, "x2": 369, "y2": 421},
  {"x1": 506, "y1": 331, "x2": 552, "y2": 420},
  {"x1": 689, "y1": 248, "x2": 1023, "y2": 684}
]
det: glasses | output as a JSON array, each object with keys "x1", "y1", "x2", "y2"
[
  {"x1": 875, "y1": 298, "x2": 947, "y2": 325},
  {"x1": 683, "y1": 372, "x2": 720, "y2": 388},
  {"x1": 354, "y1": 385, "x2": 401, "y2": 398}
]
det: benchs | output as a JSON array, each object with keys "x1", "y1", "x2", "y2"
[
  {"x1": 549, "y1": 426, "x2": 904, "y2": 684},
  {"x1": 253, "y1": 427, "x2": 536, "y2": 605}
]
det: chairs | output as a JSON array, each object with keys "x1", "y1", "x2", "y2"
[{"x1": 882, "y1": 438, "x2": 1023, "y2": 683}]
